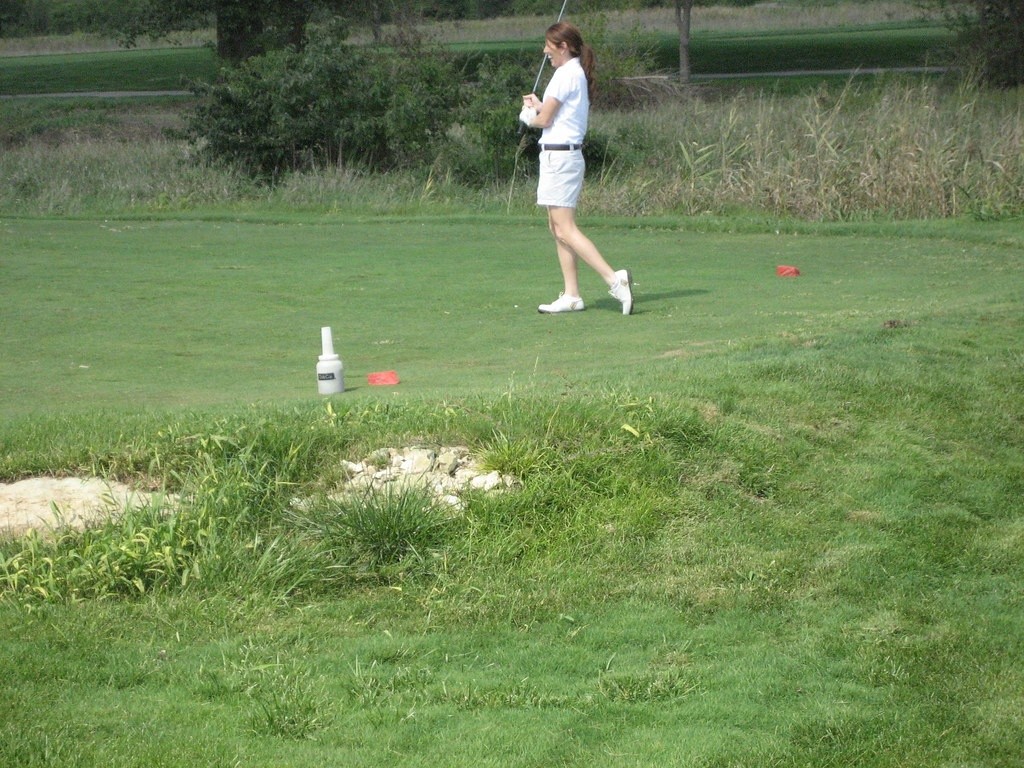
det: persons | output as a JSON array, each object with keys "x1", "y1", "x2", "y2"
[{"x1": 519, "y1": 20, "x2": 634, "y2": 315}]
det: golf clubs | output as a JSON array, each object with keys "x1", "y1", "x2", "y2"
[{"x1": 515, "y1": 0, "x2": 569, "y2": 136}]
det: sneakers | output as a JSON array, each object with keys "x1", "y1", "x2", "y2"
[
  {"x1": 538, "y1": 291, "x2": 585, "y2": 314},
  {"x1": 608, "y1": 269, "x2": 634, "y2": 315}
]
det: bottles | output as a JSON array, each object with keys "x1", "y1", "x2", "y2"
[{"x1": 316, "y1": 326, "x2": 344, "y2": 395}]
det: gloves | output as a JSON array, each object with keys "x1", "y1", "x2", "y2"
[{"x1": 519, "y1": 107, "x2": 536, "y2": 127}]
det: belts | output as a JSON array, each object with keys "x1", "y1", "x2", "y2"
[{"x1": 538, "y1": 144, "x2": 582, "y2": 150}]
